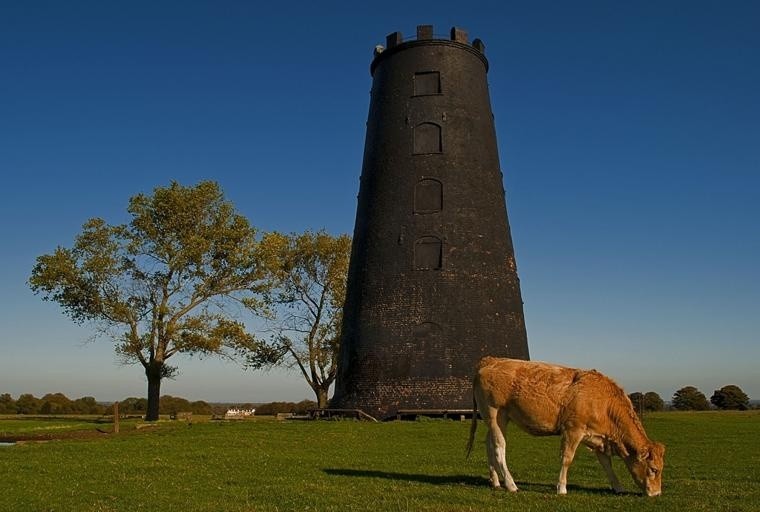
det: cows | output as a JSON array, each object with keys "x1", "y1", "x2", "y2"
[{"x1": 464, "y1": 355, "x2": 666, "y2": 498}]
